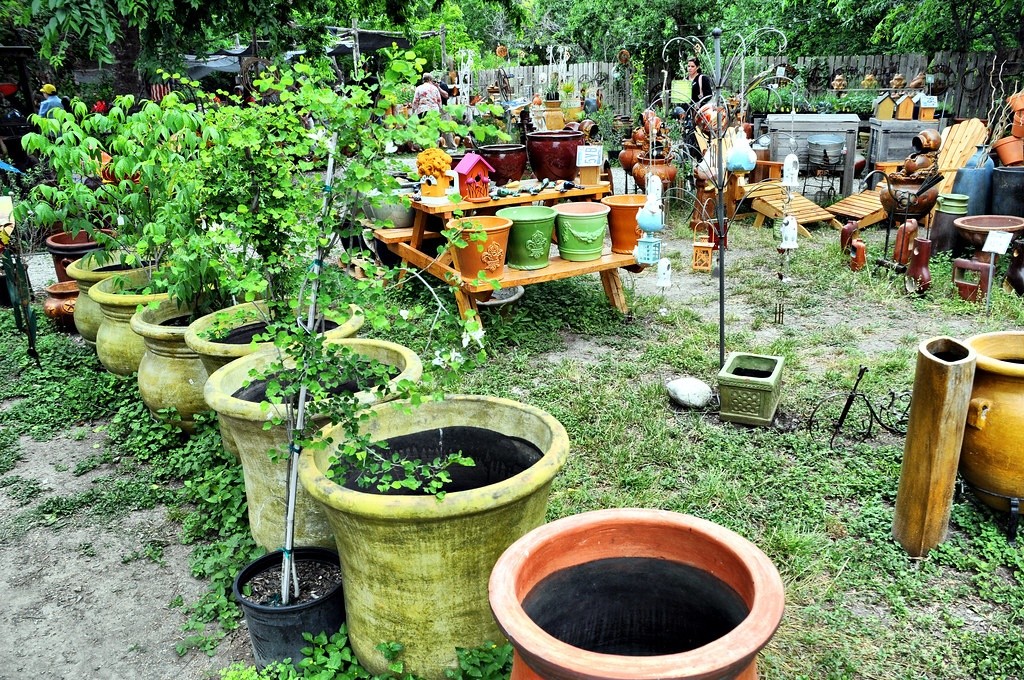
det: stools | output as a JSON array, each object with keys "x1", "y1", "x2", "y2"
[{"x1": 373, "y1": 222, "x2": 452, "y2": 244}]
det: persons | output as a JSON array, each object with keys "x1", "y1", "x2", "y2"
[
  {"x1": 675, "y1": 58, "x2": 714, "y2": 163},
  {"x1": 38, "y1": 83, "x2": 79, "y2": 139},
  {"x1": 288, "y1": 74, "x2": 459, "y2": 162}
]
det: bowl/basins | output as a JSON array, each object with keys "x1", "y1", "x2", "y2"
[{"x1": 476, "y1": 285, "x2": 525, "y2": 315}]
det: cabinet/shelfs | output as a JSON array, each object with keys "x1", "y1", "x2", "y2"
[
  {"x1": 767, "y1": 113, "x2": 860, "y2": 198},
  {"x1": 870, "y1": 117, "x2": 947, "y2": 186}
]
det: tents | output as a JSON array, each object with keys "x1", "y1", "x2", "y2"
[{"x1": 152, "y1": 23, "x2": 441, "y2": 107}]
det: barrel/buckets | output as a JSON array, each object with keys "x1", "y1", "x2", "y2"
[
  {"x1": 553, "y1": 202, "x2": 611, "y2": 261},
  {"x1": 806, "y1": 134, "x2": 846, "y2": 165},
  {"x1": 479, "y1": 145, "x2": 527, "y2": 185},
  {"x1": 526, "y1": 131, "x2": 585, "y2": 180},
  {"x1": 446, "y1": 215, "x2": 512, "y2": 283},
  {"x1": 496, "y1": 207, "x2": 558, "y2": 271},
  {"x1": 545, "y1": 108, "x2": 565, "y2": 130},
  {"x1": 600, "y1": 194, "x2": 647, "y2": 254}
]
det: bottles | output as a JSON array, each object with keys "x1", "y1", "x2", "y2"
[
  {"x1": 488, "y1": 507, "x2": 785, "y2": 680},
  {"x1": 563, "y1": 119, "x2": 599, "y2": 139},
  {"x1": 967, "y1": 145, "x2": 994, "y2": 193}
]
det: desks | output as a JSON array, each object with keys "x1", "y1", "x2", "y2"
[{"x1": 396, "y1": 179, "x2": 628, "y2": 336}]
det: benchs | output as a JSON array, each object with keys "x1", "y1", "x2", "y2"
[{"x1": 460, "y1": 251, "x2": 637, "y2": 292}]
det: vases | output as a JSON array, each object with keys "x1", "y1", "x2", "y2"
[
  {"x1": 297, "y1": 394, "x2": 569, "y2": 680},
  {"x1": 203, "y1": 341, "x2": 424, "y2": 555},
  {"x1": 335, "y1": 109, "x2": 676, "y2": 282},
  {"x1": 958, "y1": 332, "x2": 1024, "y2": 524},
  {"x1": 489, "y1": 508, "x2": 784, "y2": 680},
  {"x1": 880, "y1": 88, "x2": 1024, "y2": 299},
  {"x1": 718, "y1": 351, "x2": 785, "y2": 427},
  {"x1": 44, "y1": 280, "x2": 81, "y2": 326}
]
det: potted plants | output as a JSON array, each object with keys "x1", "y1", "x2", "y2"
[{"x1": 0, "y1": 42, "x2": 475, "y2": 680}]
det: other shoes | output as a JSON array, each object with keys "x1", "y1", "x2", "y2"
[{"x1": 446, "y1": 149, "x2": 457, "y2": 153}]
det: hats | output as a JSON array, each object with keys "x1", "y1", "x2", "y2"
[{"x1": 40, "y1": 84, "x2": 56, "y2": 95}]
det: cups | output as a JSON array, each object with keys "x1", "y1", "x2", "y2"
[{"x1": 994, "y1": 136, "x2": 1024, "y2": 166}]
